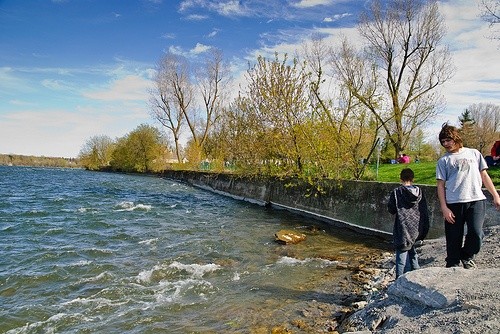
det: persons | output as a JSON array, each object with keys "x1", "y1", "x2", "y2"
[
  {"x1": 397, "y1": 154, "x2": 403, "y2": 161},
  {"x1": 484, "y1": 133, "x2": 500, "y2": 167},
  {"x1": 436, "y1": 119, "x2": 500, "y2": 268},
  {"x1": 387, "y1": 168, "x2": 427, "y2": 279},
  {"x1": 398, "y1": 154, "x2": 411, "y2": 163},
  {"x1": 414, "y1": 154, "x2": 419, "y2": 163}
]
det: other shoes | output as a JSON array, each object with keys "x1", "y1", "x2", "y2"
[{"x1": 462, "y1": 259, "x2": 477, "y2": 270}]
